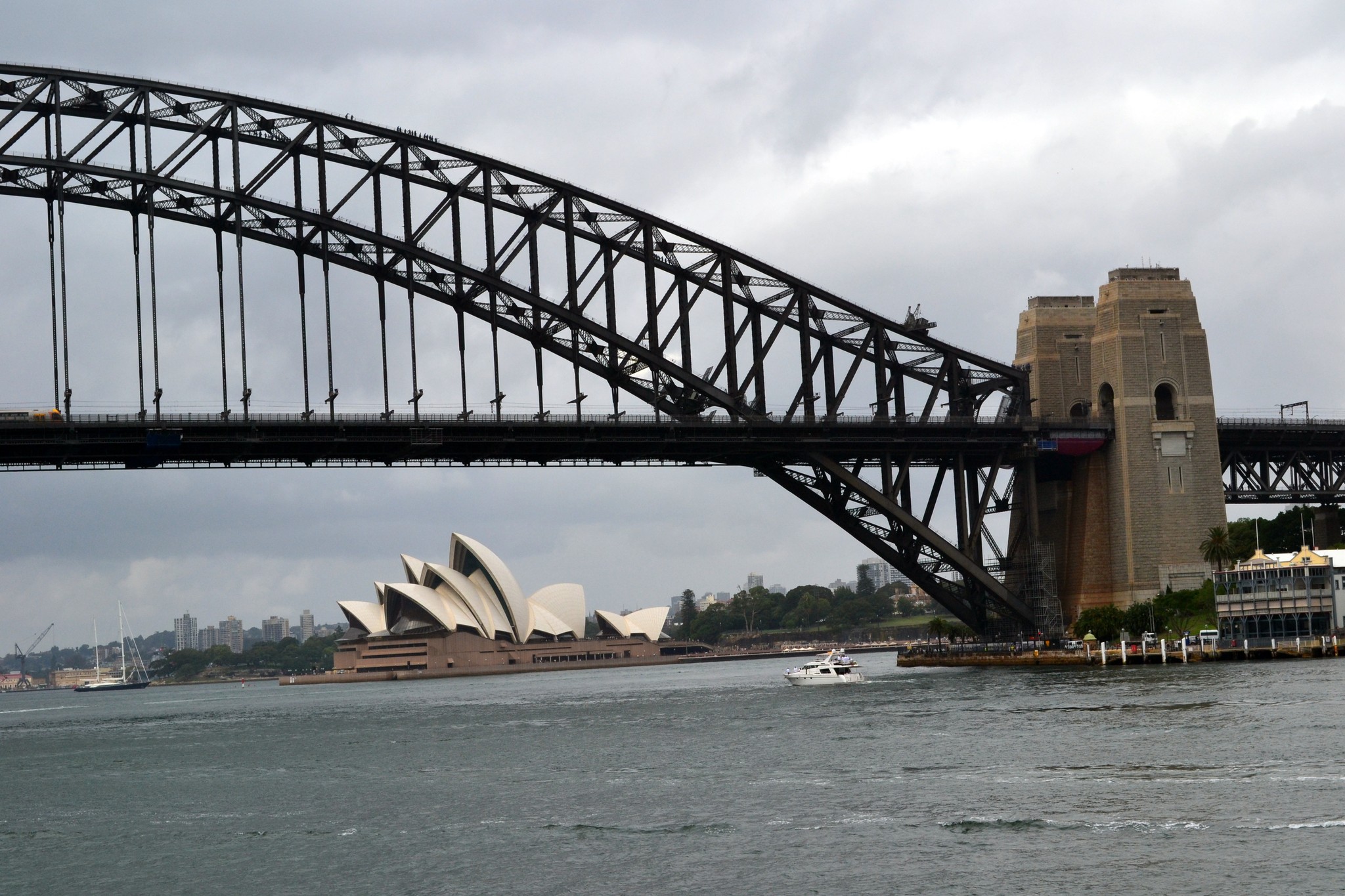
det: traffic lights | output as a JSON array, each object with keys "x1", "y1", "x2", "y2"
[{"x1": 1038, "y1": 632, "x2": 1041, "y2": 637}]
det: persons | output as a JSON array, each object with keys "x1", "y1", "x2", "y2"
[
  {"x1": 794, "y1": 666, "x2": 802, "y2": 672},
  {"x1": 1146, "y1": 638, "x2": 1159, "y2": 652},
  {"x1": 1009, "y1": 645, "x2": 1021, "y2": 652},
  {"x1": 787, "y1": 668, "x2": 790, "y2": 674},
  {"x1": 1166, "y1": 638, "x2": 1182, "y2": 651},
  {"x1": 838, "y1": 654, "x2": 850, "y2": 663}
]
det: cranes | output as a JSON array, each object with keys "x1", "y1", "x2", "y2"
[{"x1": 14, "y1": 623, "x2": 55, "y2": 689}]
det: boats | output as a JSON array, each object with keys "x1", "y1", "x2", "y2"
[{"x1": 782, "y1": 648, "x2": 865, "y2": 686}]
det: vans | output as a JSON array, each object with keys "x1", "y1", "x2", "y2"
[
  {"x1": 1199, "y1": 629, "x2": 1219, "y2": 643},
  {"x1": 1184, "y1": 636, "x2": 1199, "y2": 645},
  {"x1": 1064, "y1": 640, "x2": 1083, "y2": 650}
]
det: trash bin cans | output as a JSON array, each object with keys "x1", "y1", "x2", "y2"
[
  {"x1": 1131, "y1": 645, "x2": 1137, "y2": 652},
  {"x1": 1231, "y1": 640, "x2": 1236, "y2": 647}
]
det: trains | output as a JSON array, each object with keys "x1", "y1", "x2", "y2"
[{"x1": 0, "y1": 408, "x2": 65, "y2": 424}]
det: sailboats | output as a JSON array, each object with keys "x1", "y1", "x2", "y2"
[{"x1": 73, "y1": 599, "x2": 152, "y2": 692}]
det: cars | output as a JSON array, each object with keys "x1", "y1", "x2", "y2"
[{"x1": 1114, "y1": 643, "x2": 1120, "y2": 647}]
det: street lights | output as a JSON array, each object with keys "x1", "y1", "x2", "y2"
[
  {"x1": 1122, "y1": 628, "x2": 1124, "y2": 642},
  {"x1": 1144, "y1": 631, "x2": 1148, "y2": 651},
  {"x1": 935, "y1": 637, "x2": 938, "y2": 652},
  {"x1": 1065, "y1": 631, "x2": 1068, "y2": 642},
  {"x1": 918, "y1": 638, "x2": 921, "y2": 652},
  {"x1": 944, "y1": 637, "x2": 946, "y2": 644},
  {"x1": 1205, "y1": 624, "x2": 1208, "y2": 644},
  {"x1": 1164, "y1": 626, "x2": 1173, "y2": 652}
]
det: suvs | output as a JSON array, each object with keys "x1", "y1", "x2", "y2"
[{"x1": 337, "y1": 669, "x2": 344, "y2": 674}]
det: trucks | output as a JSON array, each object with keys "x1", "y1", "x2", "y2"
[{"x1": 1125, "y1": 633, "x2": 1155, "y2": 647}]
